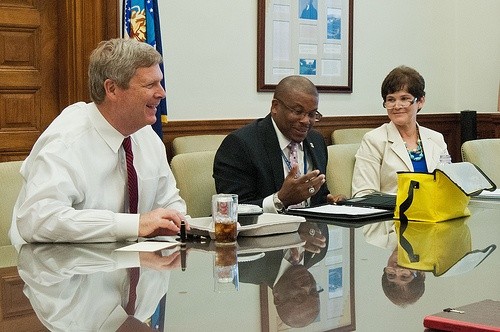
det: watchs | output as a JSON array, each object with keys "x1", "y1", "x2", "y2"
[{"x1": 273, "y1": 191, "x2": 286, "y2": 214}]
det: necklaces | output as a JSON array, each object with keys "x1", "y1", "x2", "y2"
[{"x1": 401, "y1": 127, "x2": 424, "y2": 162}]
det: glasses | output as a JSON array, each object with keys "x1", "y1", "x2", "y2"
[
  {"x1": 277, "y1": 284, "x2": 324, "y2": 305},
  {"x1": 278, "y1": 98, "x2": 322, "y2": 122},
  {"x1": 383, "y1": 267, "x2": 418, "y2": 278},
  {"x1": 383, "y1": 98, "x2": 418, "y2": 109}
]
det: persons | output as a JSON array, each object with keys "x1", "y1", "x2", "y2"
[
  {"x1": 236, "y1": 222, "x2": 330, "y2": 328},
  {"x1": 17, "y1": 248, "x2": 188, "y2": 332},
  {"x1": 362, "y1": 219, "x2": 426, "y2": 308},
  {"x1": 352, "y1": 66, "x2": 454, "y2": 198},
  {"x1": 211, "y1": 75, "x2": 345, "y2": 213},
  {"x1": 8, "y1": 39, "x2": 190, "y2": 247}
]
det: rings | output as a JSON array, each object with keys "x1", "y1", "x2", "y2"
[
  {"x1": 308, "y1": 229, "x2": 316, "y2": 237},
  {"x1": 309, "y1": 187, "x2": 316, "y2": 194}
]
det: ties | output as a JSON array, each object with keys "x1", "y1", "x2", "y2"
[
  {"x1": 123, "y1": 135, "x2": 138, "y2": 213},
  {"x1": 126, "y1": 268, "x2": 140, "y2": 315},
  {"x1": 291, "y1": 247, "x2": 301, "y2": 266},
  {"x1": 288, "y1": 141, "x2": 305, "y2": 209}
]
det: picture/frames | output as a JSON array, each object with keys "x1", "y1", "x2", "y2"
[
  {"x1": 261, "y1": 228, "x2": 356, "y2": 332},
  {"x1": 257, "y1": 0, "x2": 354, "y2": 94}
]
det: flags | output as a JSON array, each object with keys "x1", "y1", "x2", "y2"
[{"x1": 123, "y1": 0, "x2": 168, "y2": 139}]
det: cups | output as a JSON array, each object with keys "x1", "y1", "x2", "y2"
[
  {"x1": 211, "y1": 193, "x2": 238, "y2": 243},
  {"x1": 213, "y1": 246, "x2": 238, "y2": 293}
]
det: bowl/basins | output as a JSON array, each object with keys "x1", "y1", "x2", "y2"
[{"x1": 238, "y1": 212, "x2": 263, "y2": 226}]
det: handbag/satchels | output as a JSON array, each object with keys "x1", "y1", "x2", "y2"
[
  {"x1": 393, "y1": 161, "x2": 497, "y2": 223},
  {"x1": 394, "y1": 215, "x2": 497, "y2": 280}
]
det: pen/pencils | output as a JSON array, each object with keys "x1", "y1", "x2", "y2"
[{"x1": 177, "y1": 233, "x2": 207, "y2": 240}]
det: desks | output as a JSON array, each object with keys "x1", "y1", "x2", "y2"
[{"x1": 0, "y1": 199, "x2": 500, "y2": 332}]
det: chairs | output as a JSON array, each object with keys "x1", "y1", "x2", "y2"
[
  {"x1": 170, "y1": 135, "x2": 227, "y2": 217},
  {"x1": 461, "y1": 138, "x2": 500, "y2": 189},
  {"x1": 0, "y1": 161, "x2": 24, "y2": 246},
  {"x1": 326, "y1": 128, "x2": 375, "y2": 199}
]
